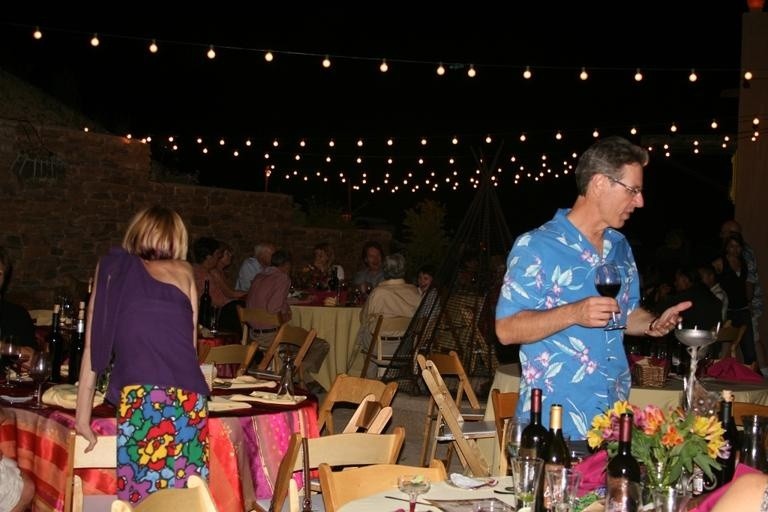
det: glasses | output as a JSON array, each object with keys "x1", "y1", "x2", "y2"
[{"x1": 590, "y1": 170, "x2": 644, "y2": 197}]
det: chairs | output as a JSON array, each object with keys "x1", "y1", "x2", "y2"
[
  {"x1": 421, "y1": 360, "x2": 498, "y2": 477},
  {"x1": 255, "y1": 323, "x2": 317, "y2": 391},
  {"x1": 253, "y1": 425, "x2": 406, "y2": 512},
  {"x1": 361, "y1": 314, "x2": 425, "y2": 381},
  {"x1": 342, "y1": 392, "x2": 393, "y2": 433},
  {"x1": 316, "y1": 374, "x2": 398, "y2": 435},
  {"x1": 716, "y1": 400, "x2": 768, "y2": 470},
  {"x1": 318, "y1": 459, "x2": 448, "y2": 511},
  {"x1": 237, "y1": 305, "x2": 284, "y2": 371},
  {"x1": 421, "y1": 350, "x2": 486, "y2": 466},
  {"x1": 109, "y1": 473, "x2": 217, "y2": 512},
  {"x1": 491, "y1": 389, "x2": 520, "y2": 447},
  {"x1": 0, "y1": 408, "x2": 74, "y2": 512},
  {"x1": 202, "y1": 341, "x2": 258, "y2": 377}
]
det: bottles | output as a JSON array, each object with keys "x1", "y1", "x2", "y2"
[
  {"x1": 536, "y1": 406, "x2": 571, "y2": 512},
  {"x1": 710, "y1": 389, "x2": 738, "y2": 488},
  {"x1": 45, "y1": 304, "x2": 64, "y2": 382},
  {"x1": 199, "y1": 280, "x2": 211, "y2": 329},
  {"x1": 518, "y1": 389, "x2": 549, "y2": 512},
  {"x1": 604, "y1": 414, "x2": 640, "y2": 512},
  {"x1": 68, "y1": 302, "x2": 87, "y2": 385}
]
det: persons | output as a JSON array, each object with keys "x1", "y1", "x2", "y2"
[
  {"x1": 211, "y1": 245, "x2": 246, "y2": 305},
  {"x1": 496, "y1": 135, "x2": 693, "y2": 470},
  {"x1": 235, "y1": 241, "x2": 277, "y2": 310},
  {"x1": 189, "y1": 238, "x2": 245, "y2": 343},
  {"x1": 349, "y1": 253, "x2": 423, "y2": 380},
  {"x1": 414, "y1": 265, "x2": 442, "y2": 295},
  {"x1": 302, "y1": 243, "x2": 352, "y2": 290},
  {"x1": 0, "y1": 251, "x2": 42, "y2": 384},
  {"x1": 352, "y1": 240, "x2": 387, "y2": 287},
  {"x1": 711, "y1": 230, "x2": 763, "y2": 379},
  {"x1": 244, "y1": 250, "x2": 330, "y2": 387},
  {"x1": 650, "y1": 267, "x2": 723, "y2": 366},
  {"x1": 71, "y1": 204, "x2": 212, "y2": 511},
  {"x1": 719, "y1": 219, "x2": 768, "y2": 378},
  {"x1": 696, "y1": 264, "x2": 729, "y2": 363},
  {"x1": 710, "y1": 256, "x2": 725, "y2": 277}
]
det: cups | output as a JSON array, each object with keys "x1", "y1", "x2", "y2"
[
  {"x1": 505, "y1": 418, "x2": 533, "y2": 491},
  {"x1": 26, "y1": 348, "x2": 54, "y2": 410},
  {"x1": 0, "y1": 334, "x2": 20, "y2": 390},
  {"x1": 593, "y1": 261, "x2": 627, "y2": 331},
  {"x1": 397, "y1": 476, "x2": 430, "y2": 512},
  {"x1": 548, "y1": 469, "x2": 579, "y2": 508},
  {"x1": 512, "y1": 456, "x2": 544, "y2": 511}
]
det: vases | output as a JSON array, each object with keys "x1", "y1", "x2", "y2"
[{"x1": 650, "y1": 486, "x2": 688, "y2": 511}]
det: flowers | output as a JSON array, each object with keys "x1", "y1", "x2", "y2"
[{"x1": 586, "y1": 400, "x2": 729, "y2": 494}]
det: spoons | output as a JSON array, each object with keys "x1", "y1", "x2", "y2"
[{"x1": 450, "y1": 472, "x2": 495, "y2": 490}]
